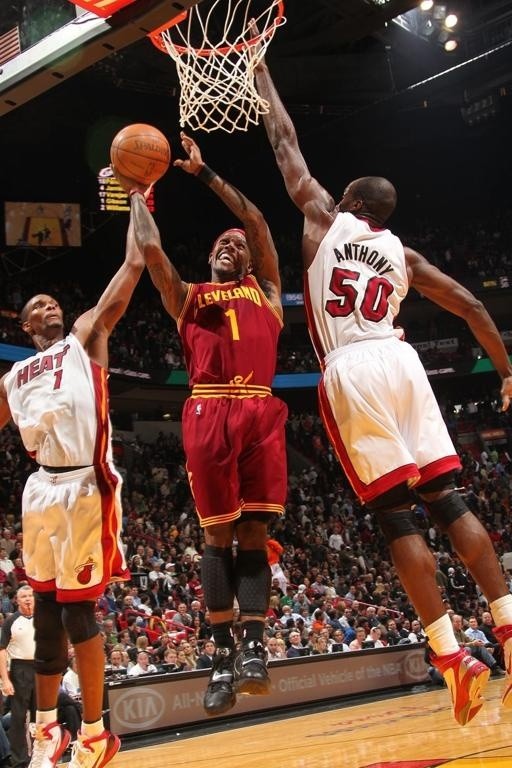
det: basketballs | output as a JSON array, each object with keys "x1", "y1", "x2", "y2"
[{"x1": 111, "y1": 124, "x2": 170, "y2": 183}]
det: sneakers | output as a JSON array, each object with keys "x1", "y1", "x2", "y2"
[
  {"x1": 26, "y1": 721, "x2": 71, "y2": 767},
  {"x1": 204, "y1": 640, "x2": 271, "y2": 716},
  {"x1": 428, "y1": 647, "x2": 491, "y2": 727},
  {"x1": 70, "y1": 729, "x2": 120, "y2": 768},
  {"x1": 491, "y1": 624, "x2": 512, "y2": 709}
]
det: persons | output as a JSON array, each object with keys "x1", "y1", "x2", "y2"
[
  {"x1": 110, "y1": 131, "x2": 284, "y2": 716},
  {"x1": 0, "y1": 580, "x2": 107, "y2": 768},
  {"x1": 0, "y1": 181, "x2": 185, "y2": 768},
  {"x1": 245, "y1": 15, "x2": 511, "y2": 727},
  {"x1": 1, "y1": 301, "x2": 476, "y2": 380},
  {"x1": 0, "y1": 394, "x2": 510, "y2": 702}
]
